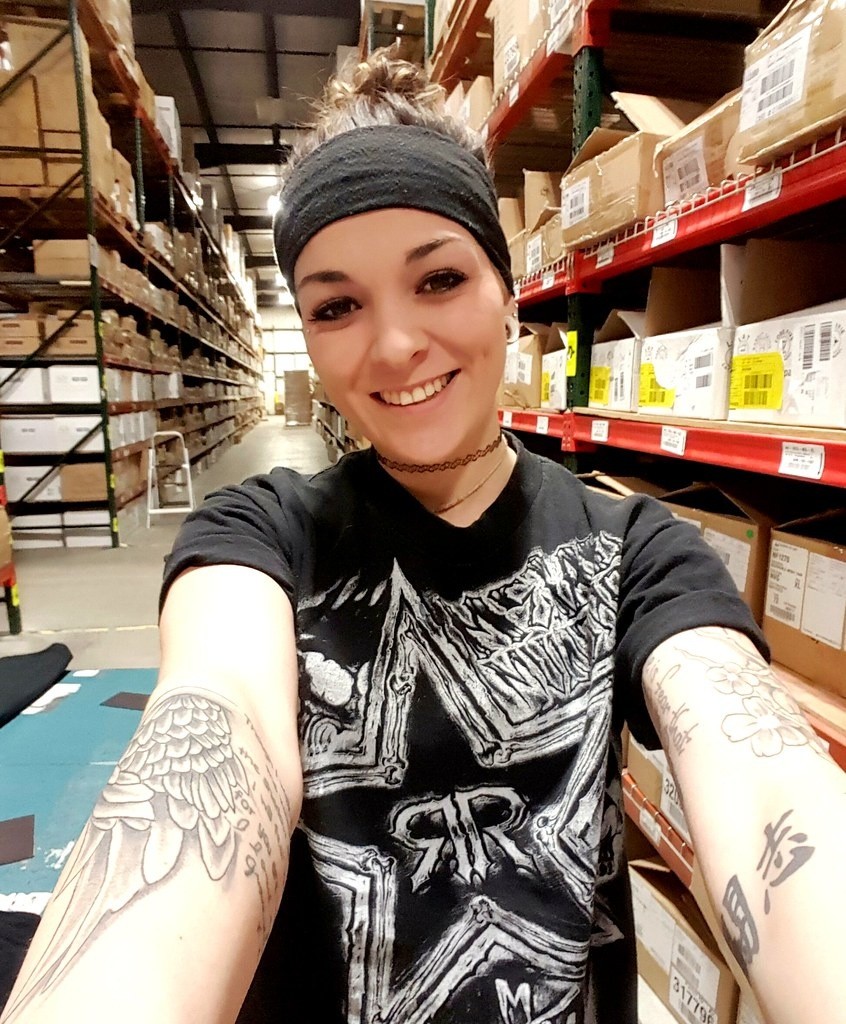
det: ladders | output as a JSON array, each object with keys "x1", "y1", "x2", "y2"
[{"x1": 146, "y1": 431, "x2": 197, "y2": 529}]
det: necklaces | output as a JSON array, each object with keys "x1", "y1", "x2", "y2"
[
  {"x1": 370, "y1": 430, "x2": 503, "y2": 473},
  {"x1": 428, "y1": 458, "x2": 504, "y2": 513}
]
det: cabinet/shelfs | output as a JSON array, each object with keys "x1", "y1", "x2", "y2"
[
  {"x1": 0, "y1": 1, "x2": 224, "y2": 547},
  {"x1": 200, "y1": 231, "x2": 265, "y2": 453},
  {"x1": 313, "y1": 397, "x2": 358, "y2": 454},
  {"x1": 416, "y1": 0, "x2": 846, "y2": 1022}
]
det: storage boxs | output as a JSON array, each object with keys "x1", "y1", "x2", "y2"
[
  {"x1": 334, "y1": 0, "x2": 422, "y2": 77},
  {"x1": 0, "y1": 1, "x2": 266, "y2": 546},
  {"x1": 282, "y1": 366, "x2": 362, "y2": 464},
  {"x1": 420, "y1": 0, "x2": 846, "y2": 1024}
]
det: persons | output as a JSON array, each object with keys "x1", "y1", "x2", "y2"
[{"x1": 0, "y1": 38, "x2": 845, "y2": 1024}]
